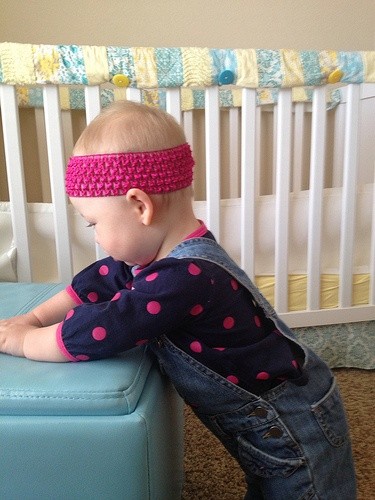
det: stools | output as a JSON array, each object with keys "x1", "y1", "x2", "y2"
[{"x1": 1, "y1": 281, "x2": 185, "y2": 500}]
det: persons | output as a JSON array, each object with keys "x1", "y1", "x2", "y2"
[{"x1": 1, "y1": 99, "x2": 357, "y2": 500}]
